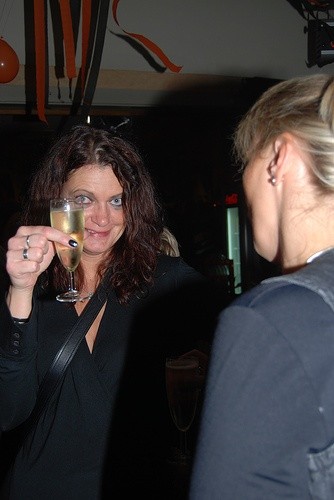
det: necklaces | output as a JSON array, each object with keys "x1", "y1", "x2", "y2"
[{"x1": 80, "y1": 290, "x2": 93, "y2": 305}]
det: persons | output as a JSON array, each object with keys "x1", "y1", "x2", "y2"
[
  {"x1": 0, "y1": 128, "x2": 213, "y2": 499},
  {"x1": 188, "y1": 63, "x2": 334, "y2": 499}
]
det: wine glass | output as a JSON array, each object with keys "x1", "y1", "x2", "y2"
[
  {"x1": 164, "y1": 357, "x2": 198, "y2": 464},
  {"x1": 50, "y1": 199, "x2": 93, "y2": 302}
]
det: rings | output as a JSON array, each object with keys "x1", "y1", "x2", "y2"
[
  {"x1": 22, "y1": 249, "x2": 29, "y2": 260},
  {"x1": 26, "y1": 235, "x2": 31, "y2": 248}
]
